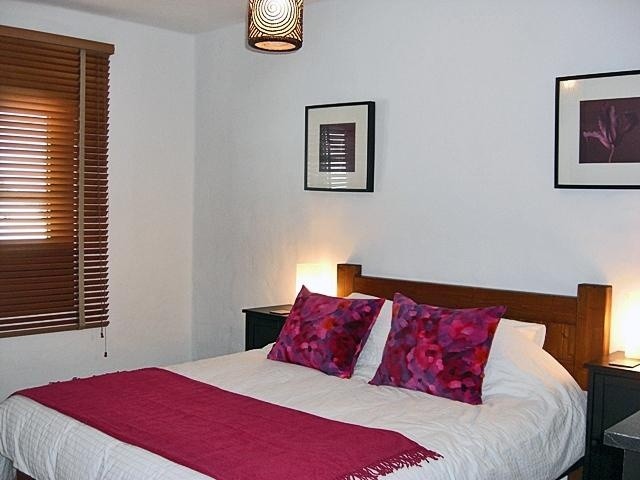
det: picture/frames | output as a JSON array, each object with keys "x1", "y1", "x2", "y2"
[
  {"x1": 555, "y1": 70, "x2": 640, "y2": 189},
  {"x1": 304, "y1": 102, "x2": 375, "y2": 192}
]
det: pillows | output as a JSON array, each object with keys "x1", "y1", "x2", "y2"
[{"x1": 264, "y1": 284, "x2": 546, "y2": 404}]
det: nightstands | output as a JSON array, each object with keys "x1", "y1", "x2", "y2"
[
  {"x1": 242, "y1": 304, "x2": 293, "y2": 351},
  {"x1": 583, "y1": 351, "x2": 640, "y2": 480}
]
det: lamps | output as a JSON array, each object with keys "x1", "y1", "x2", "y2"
[
  {"x1": 295, "y1": 264, "x2": 338, "y2": 300},
  {"x1": 608, "y1": 290, "x2": 640, "y2": 369},
  {"x1": 248, "y1": 0, "x2": 303, "y2": 53}
]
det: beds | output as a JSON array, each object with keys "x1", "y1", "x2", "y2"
[{"x1": 0, "y1": 264, "x2": 612, "y2": 480}]
